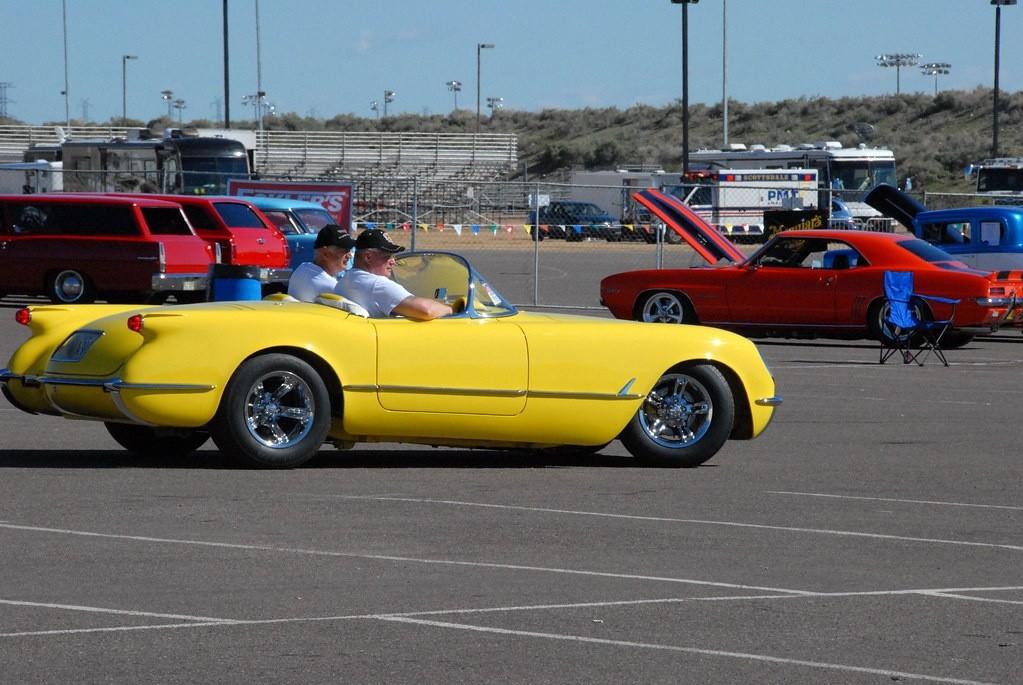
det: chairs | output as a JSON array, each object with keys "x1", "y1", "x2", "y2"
[
  {"x1": 312, "y1": 294, "x2": 371, "y2": 318},
  {"x1": 263, "y1": 293, "x2": 300, "y2": 302},
  {"x1": 833, "y1": 254, "x2": 850, "y2": 269},
  {"x1": 878, "y1": 270, "x2": 961, "y2": 367}
]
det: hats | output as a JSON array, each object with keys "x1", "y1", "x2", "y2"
[
  {"x1": 314, "y1": 224, "x2": 357, "y2": 249},
  {"x1": 356, "y1": 228, "x2": 406, "y2": 253}
]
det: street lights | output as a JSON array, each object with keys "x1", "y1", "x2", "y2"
[
  {"x1": 122, "y1": 55, "x2": 137, "y2": 127},
  {"x1": 476, "y1": 45, "x2": 495, "y2": 132}
]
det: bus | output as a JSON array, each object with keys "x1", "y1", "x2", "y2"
[{"x1": 688, "y1": 141, "x2": 898, "y2": 217}]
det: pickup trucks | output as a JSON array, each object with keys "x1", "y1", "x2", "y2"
[{"x1": 526, "y1": 201, "x2": 623, "y2": 242}]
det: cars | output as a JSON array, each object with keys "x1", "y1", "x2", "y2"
[
  {"x1": 0, "y1": 195, "x2": 217, "y2": 305},
  {"x1": 0, "y1": 251, "x2": 782, "y2": 470},
  {"x1": 831, "y1": 198, "x2": 860, "y2": 230},
  {"x1": 599, "y1": 189, "x2": 1023, "y2": 347},
  {"x1": 33, "y1": 192, "x2": 293, "y2": 283},
  {"x1": 192, "y1": 195, "x2": 357, "y2": 281},
  {"x1": 864, "y1": 181, "x2": 1022, "y2": 272}
]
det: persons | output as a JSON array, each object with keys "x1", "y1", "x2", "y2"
[
  {"x1": 333, "y1": 228, "x2": 452, "y2": 321},
  {"x1": 288, "y1": 224, "x2": 355, "y2": 302}
]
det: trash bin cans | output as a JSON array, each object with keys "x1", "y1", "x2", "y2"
[{"x1": 210, "y1": 263, "x2": 262, "y2": 302}]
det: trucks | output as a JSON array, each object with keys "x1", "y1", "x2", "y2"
[
  {"x1": 636, "y1": 167, "x2": 818, "y2": 245},
  {"x1": 965, "y1": 157, "x2": 1023, "y2": 207},
  {"x1": 62, "y1": 127, "x2": 261, "y2": 195}
]
